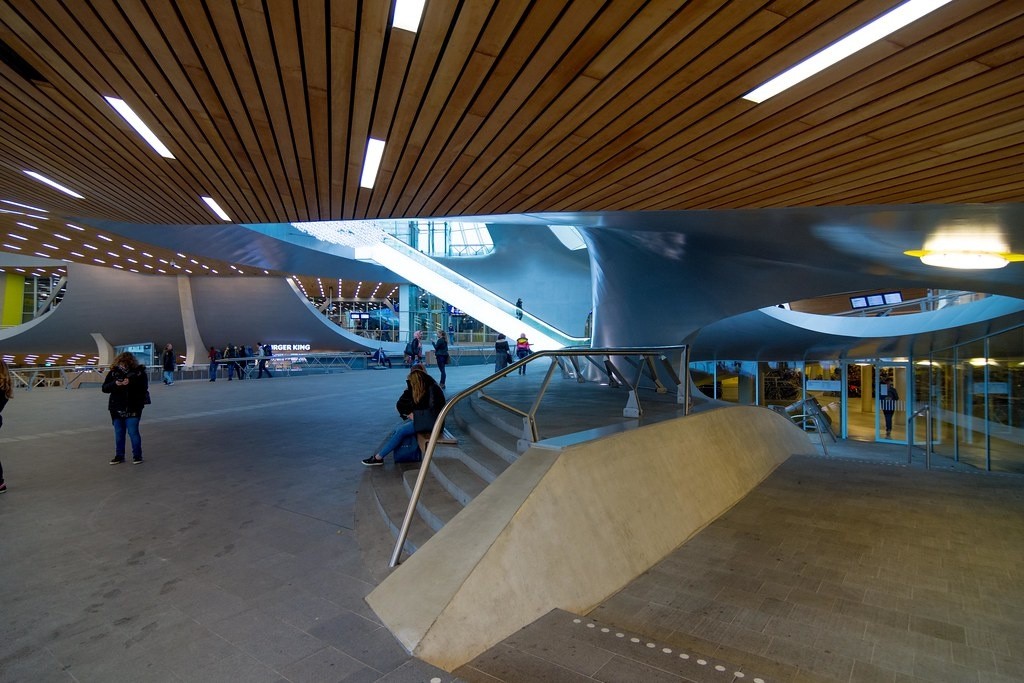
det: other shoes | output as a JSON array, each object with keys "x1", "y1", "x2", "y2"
[
  {"x1": 133, "y1": 456, "x2": 143, "y2": 465},
  {"x1": 109, "y1": 455, "x2": 125, "y2": 465},
  {"x1": 439, "y1": 383, "x2": 445, "y2": 389},
  {"x1": 0, "y1": 483, "x2": 7, "y2": 494}
]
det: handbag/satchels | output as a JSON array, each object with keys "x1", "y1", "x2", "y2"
[
  {"x1": 445, "y1": 355, "x2": 451, "y2": 364},
  {"x1": 133, "y1": 364, "x2": 151, "y2": 405},
  {"x1": 404, "y1": 339, "x2": 419, "y2": 356},
  {"x1": 506, "y1": 349, "x2": 512, "y2": 364},
  {"x1": 393, "y1": 433, "x2": 422, "y2": 464}
]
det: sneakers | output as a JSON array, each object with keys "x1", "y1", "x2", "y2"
[{"x1": 361, "y1": 455, "x2": 385, "y2": 466}]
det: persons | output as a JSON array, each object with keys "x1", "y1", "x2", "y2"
[
  {"x1": 101, "y1": 352, "x2": 152, "y2": 464},
  {"x1": 515, "y1": 298, "x2": 523, "y2": 321},
  {"x1": 357, "y1": 321, "x2": 392, "y2": 342},
  {"x1": 880, "y1": 379, "x2": 900, "y2": 438},
  {"x1": 405, "y1": 330, "x2": 427, "y2": 372},
  {"x1": 362, "y1": 364, "x2": 446, "y2": 466},
  {"x1": 516, "y1": 333, "x2": 532, "y2": 377},
  {"x1": 161, "y1": 343, "x2": 176, "y2": 386},
  {"x1": 374, "y1": 347, "x2": 392, "y2": 369},
  {"x1": 0, "y1": 359, "x2": 13, "y2": 493},
  {"x1": 223, "y1": 342, "x2": 273, "y2": 382},
  {"x1": 449, "y1": 323, "x2": 455, "y2": 346},
  {"x1": 363, "y1": 352, "x2": 372, "y2": 360},
  {"x1": 208, "y1": 346, "x2": 218, "y2": 382},
  {"x1": 495, "y1": 334, "x2": 510, "y2": 377},
  {"x1": 430, "y1": 330, "x2": 449, "y2": 390}
]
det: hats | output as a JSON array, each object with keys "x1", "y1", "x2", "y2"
[{"x1": 410, "y1": 364, "x2": 426, "y2": 374}]
